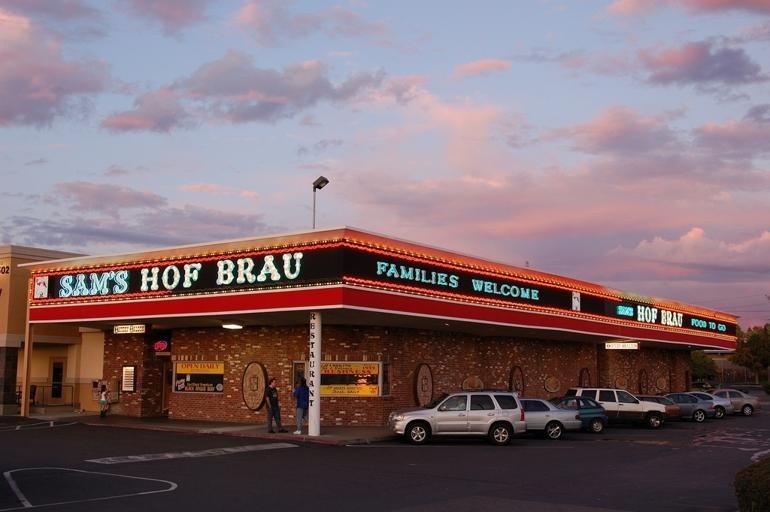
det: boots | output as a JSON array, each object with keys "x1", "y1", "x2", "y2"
[{"x1": 101, "y1": 409, "x2": 107, "y2": 417}]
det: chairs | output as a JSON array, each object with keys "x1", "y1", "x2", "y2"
[{"x1": 17, "y1": 384, "x2": 37, "y2": 406}]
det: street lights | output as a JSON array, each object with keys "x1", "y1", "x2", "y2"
[{"x1": 312, "y1": 176, "x2": 329, "y2": 229}]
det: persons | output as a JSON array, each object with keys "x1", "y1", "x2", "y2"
[
  {"x1": 99, "y1": 384, "x2": 108, "y2": 415},
  {"x1": 291, "y1": 378, "x2": 309, "y2": 434},
  {"x1": 264, "y1": 378, "x2": 288, "y2": 433}
]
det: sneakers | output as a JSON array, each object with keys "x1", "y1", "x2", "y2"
[
  {"x1": 293, "y1": 430, "x2": 301, "y2": 434},
  {"x1": 269, "y1": 429, "x2": 288, "y2": 433}
]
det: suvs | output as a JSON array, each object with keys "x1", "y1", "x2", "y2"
[
  {"x1": 564, "y1": 387, "x2": 668, "y2": 429},
  {"x1": 388, "y1": 388, "x2": 526, "y2": 445}
]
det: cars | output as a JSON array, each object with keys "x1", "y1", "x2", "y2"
[
  {"x1": 519, "y1": 399, "x2": 582, "y2": 440},
  {"x1": 550, "y1": 396, "x2": 608, "y2": 434},
  {"x1": 636, "y1": 388, "x2": 761, "y2": 423}
]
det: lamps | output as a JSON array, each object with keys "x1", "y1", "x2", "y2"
[{"x1": 221, "y1": 319, "x2": 245, "y2": 329}]
